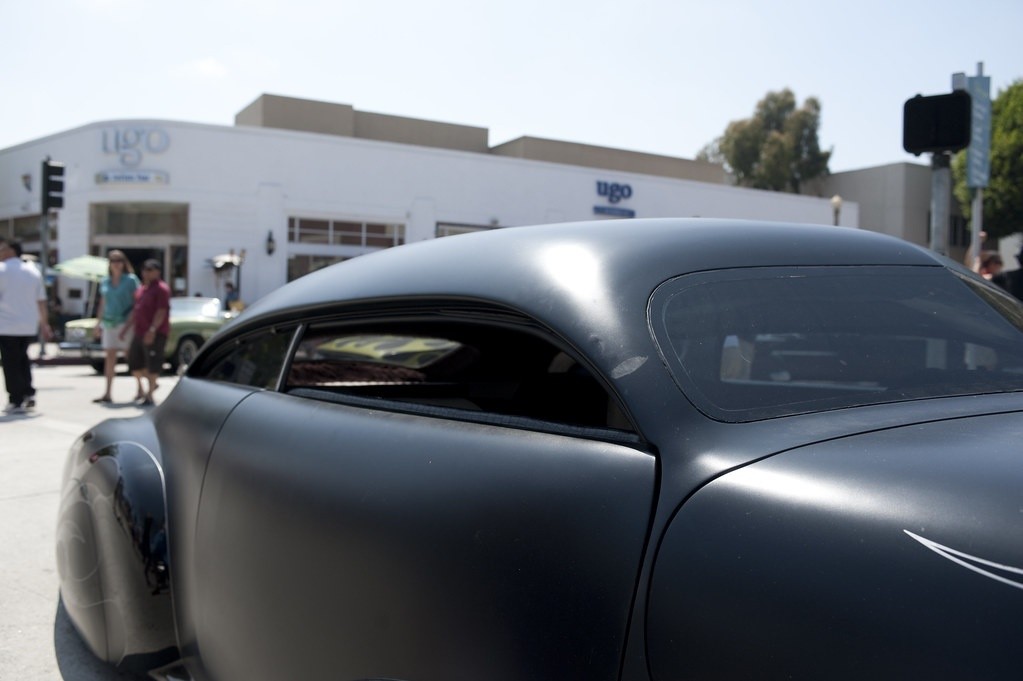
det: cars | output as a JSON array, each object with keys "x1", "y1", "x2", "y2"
[{"x1": 48, "y1": 216, "x2": 1023, "y2": 681}]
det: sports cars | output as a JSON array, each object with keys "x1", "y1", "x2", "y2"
[{"x1": 57, "y1": 296, "x2": 240, "y2": 376}]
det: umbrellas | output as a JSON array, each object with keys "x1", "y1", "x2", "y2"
[{"x1": 53, "y1": 254, "x2": 111, "y2": 319}]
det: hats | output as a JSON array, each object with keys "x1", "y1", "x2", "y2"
[
  {"x1": 0, "y1": 236, "x2": 22, "y2": 258},
  {"x1": 142, "y1": 259, "x2": 161, "y2": 269}
]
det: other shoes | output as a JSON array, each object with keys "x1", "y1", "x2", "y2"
[{"x1": 2, "y1": 400, "x2": 35, "y2": 413}]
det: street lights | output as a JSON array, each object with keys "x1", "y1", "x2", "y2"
[{"x1": 828, "y1": 194, "x2": 843, "y2": 225}]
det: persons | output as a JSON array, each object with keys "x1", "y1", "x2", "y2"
[
  {"x1": 119, "y1": 259, "x2": 172, "y2": 405},
  {"x1": 94, "y1": 249, "x2": 146, "y2": 403},
  {"x1": 965, "y1": 229, "x2": 1023, "y2": 301},
  {"x1": 0, "y1": 237, "x2": 55, "y2": 414},
  {"x1": 225, "y1": 282, "x2": 238, "y2": 311}
]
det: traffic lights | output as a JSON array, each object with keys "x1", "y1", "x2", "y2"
[
  {"x1": 39, "y1": 158, "x2": 66, "y2": 217},
  {"x1": 902, "y1": 89, "x2": 973, "y2": 157}
]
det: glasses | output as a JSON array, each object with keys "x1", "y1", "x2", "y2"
[{"x1": 110, "y1": 258, "x2": 123, "y2": 262}]
